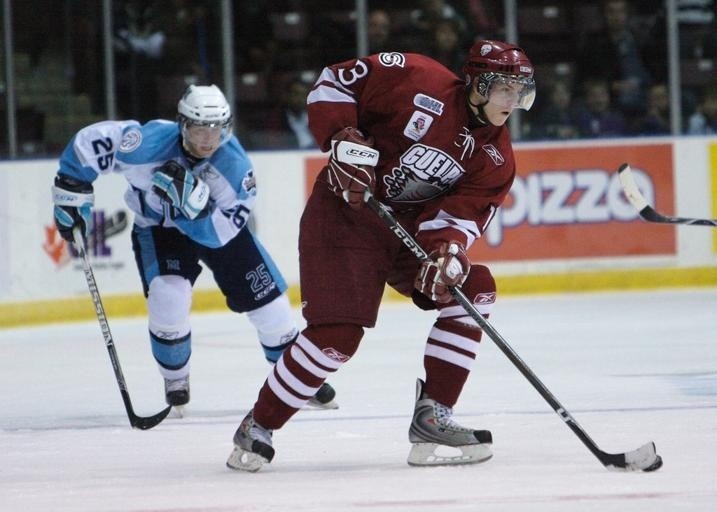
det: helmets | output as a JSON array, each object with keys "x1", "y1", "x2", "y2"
[
  {"x1": 177, "y1": 84, "x2": 230, "y2": 127},
  {"x1": 461, "y1": 40, "x2": 535, "y2": 82}
]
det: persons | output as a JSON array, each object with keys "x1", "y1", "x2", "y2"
[
  {"x1": 54, "y1": 82, "x2": 334, "y2": 405},
  {"x1": 1, "y1": 0, "x2": 716, "y2": 161},
  {"x1": 233, "y1": 38, "x2": 537, "y2": 463}
]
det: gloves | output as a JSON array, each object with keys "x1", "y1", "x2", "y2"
[
  {"x1": 414, "y1": 240, "x2": 471, "y2": 303},
  {"x1": 150, "y1": 161, "x2": 212, "y2": 223},
  {"x1": 326, "y1": 127, "x2": 379, "y2": 211},
  {"x1": 53, "y1": 171, "x2": 94, "y2": 241}
]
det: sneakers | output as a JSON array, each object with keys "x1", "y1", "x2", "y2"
[
  {"x1": 409, "y1": 379, "x2": 492, "y2": 447},
  {"x1": 316, "y1": 383, "x2": 336, "y2": 404},
  {"x1": 164, "y1": 374, "x2": 190, "y2": 406},
  {"x1": 233, "y1": 408, "x2": 275, "y2": 463}
]
